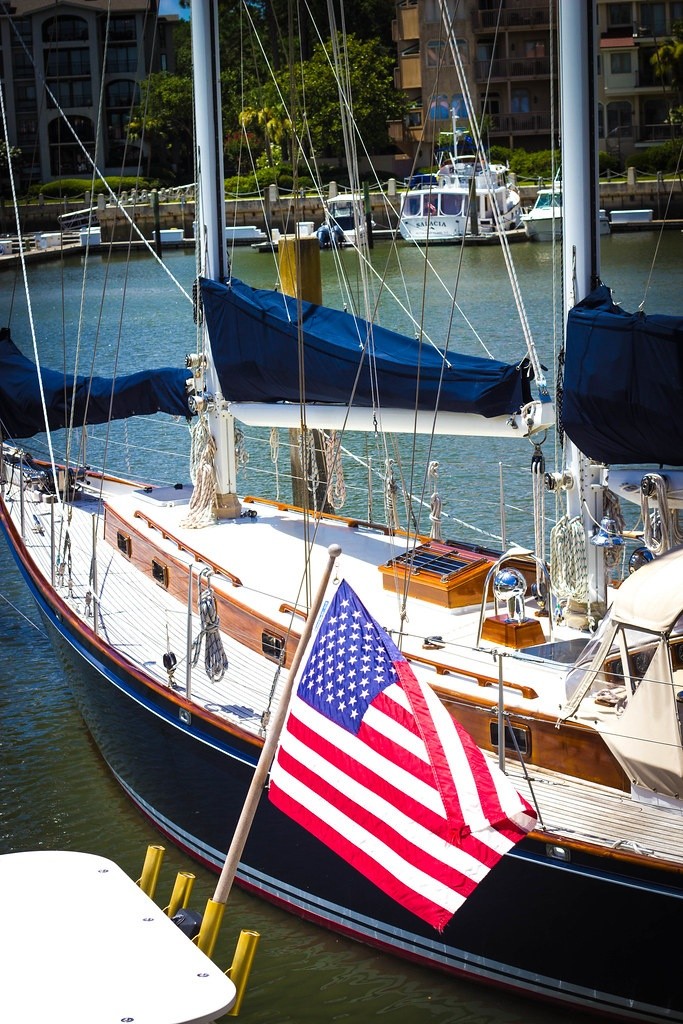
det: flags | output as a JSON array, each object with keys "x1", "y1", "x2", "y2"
[{"x1": 267, "y1": 577, "x2": 538, "y2": 934}]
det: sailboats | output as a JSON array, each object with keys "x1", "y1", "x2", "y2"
[{"x1": 0, "y1": 0, "x2": 682, "y2": 1024}]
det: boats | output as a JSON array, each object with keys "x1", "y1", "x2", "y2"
[
  {"x1": 520, "y1": 163, "x2": 612, "y2": 243},
  {"x1": 396, "y1": 108, "x2": 526, "y2": 246},
  {"x1": 316, "y1": 192, "x2": 378, "y2": 248}
]
